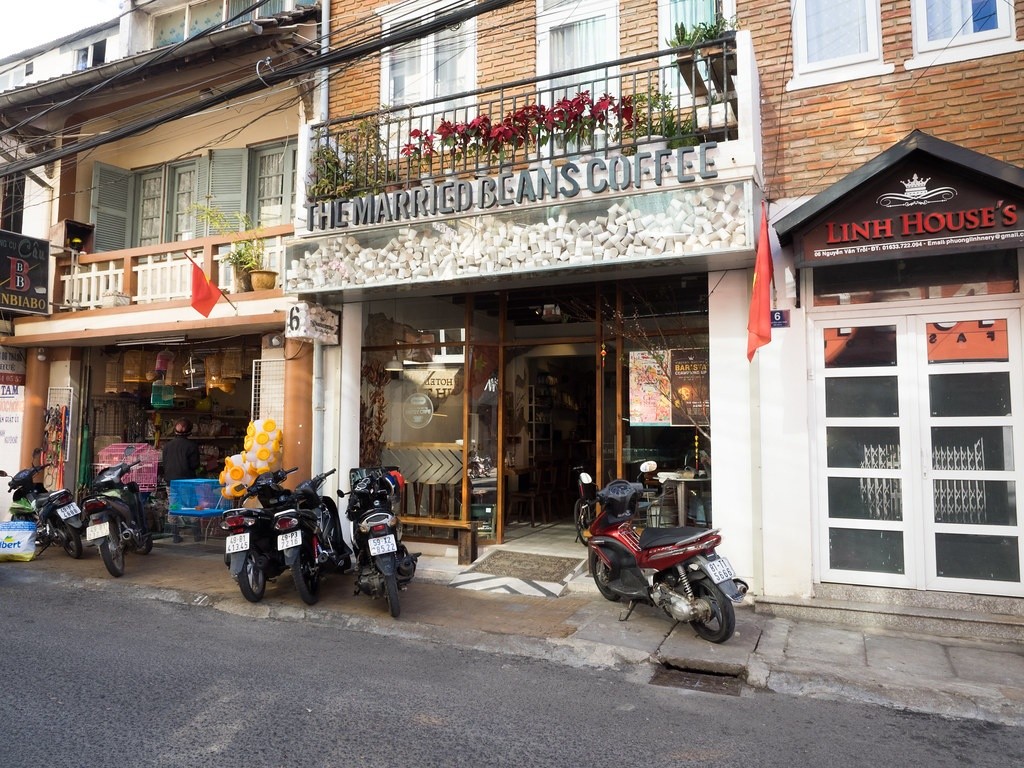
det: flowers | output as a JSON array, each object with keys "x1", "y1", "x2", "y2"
[
  {"x1": 406, "y1": 129, "x2": 439, "y2": 172},
  {"x1": 439, "y1": 93, "x2": 639, "y2": 169}
]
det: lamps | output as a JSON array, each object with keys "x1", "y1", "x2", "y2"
[{"x1": 384, "y1": 338, "x2": 404, "y2": 372}]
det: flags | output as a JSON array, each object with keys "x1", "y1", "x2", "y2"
[
  {"x1": 190, "y1": 261, "x2": 223, "y2": 318},
  {"x1": 747, "y1": 200, "x2": 773, "y2": 364}
]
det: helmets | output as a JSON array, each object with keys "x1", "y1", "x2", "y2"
[
  {"x1": 174, "y1": 418, "x2": 192, "y2": 438},
  {"x1": 390, "y1": 470, "x2": 406, "y2": 488}
]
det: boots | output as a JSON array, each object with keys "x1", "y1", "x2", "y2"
[
  {"x1": 172, "y1": 526, "x2": 183, "y2": 543},
  {"x1": 191, "y1": 522, "x2": 204, "y2": 542}
]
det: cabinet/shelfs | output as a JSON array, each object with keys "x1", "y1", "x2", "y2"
[
  {"x1": 146, "y1": 410, "x2": 249, "y2": 489},
  {"x1": 529, "y1": 383, "x2": 553, "y2": 467}
]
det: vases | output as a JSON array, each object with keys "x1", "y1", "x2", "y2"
[
  {"x1": 419, "y1": 171, "x2": 435, "y2": 187},
  {"x1": 474, "y1": 163, "x2": 489, "y2": 177},
  {"x1": 528, "y1": 154, "x2": 543, "y2": 169},
  {"x1": 499, "y1": 159, "x2": 512, "y2": 173},
  {"x1": 444, "y1": 168, "x2": 459, "y2": 185}
]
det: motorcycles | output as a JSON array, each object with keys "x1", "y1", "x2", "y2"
[
  {"x1": 336, "y1": 462, "x2": 423, "y2": 619},
  {"x1": 0, "y1": 444, "x2": 83, "y2": 559},
  {"x1": 571, "y1": 457, "x2": 748, "y2": 644},
  {"x1": 219, "y1": 463, "x2": 297, "y2": 606},
  {"x1": 81, "y1": 457, "x2": 154, "y2": 580},
  {"x1": 271, "y1": 467, "x2": 353, "y2": 608}
]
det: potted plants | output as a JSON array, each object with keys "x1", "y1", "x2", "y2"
[
  {"x1": 671, "y1": 19, "x2": 725, "y2": 56},
  {"x1": 231, "y1": 217, "x2": 277, "y2": 292},
  {"x1": 382, "y1": 168, "x2": 402, "y2": 193},
  {"x1": 628, "y1": 91, "x2": 672, "y2": 155}
]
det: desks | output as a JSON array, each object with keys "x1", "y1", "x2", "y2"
[
  {"x1": 654, "y1": 477, "x2": 711, "y2": 527},
  {"x1": 502, "y1": 465, "x2": 533, "y2": 523}
]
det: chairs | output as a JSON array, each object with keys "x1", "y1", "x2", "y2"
[
  {"x1": 504, "y1": 463, "x2": 578, "y2": 528},
  {"x1": 609, "y1": 471, "x2": 650, "y2": 528}
]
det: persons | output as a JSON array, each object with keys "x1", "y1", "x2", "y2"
[{"x1": 161, "y1": 418, "x2": 204, "y2": 544}]
